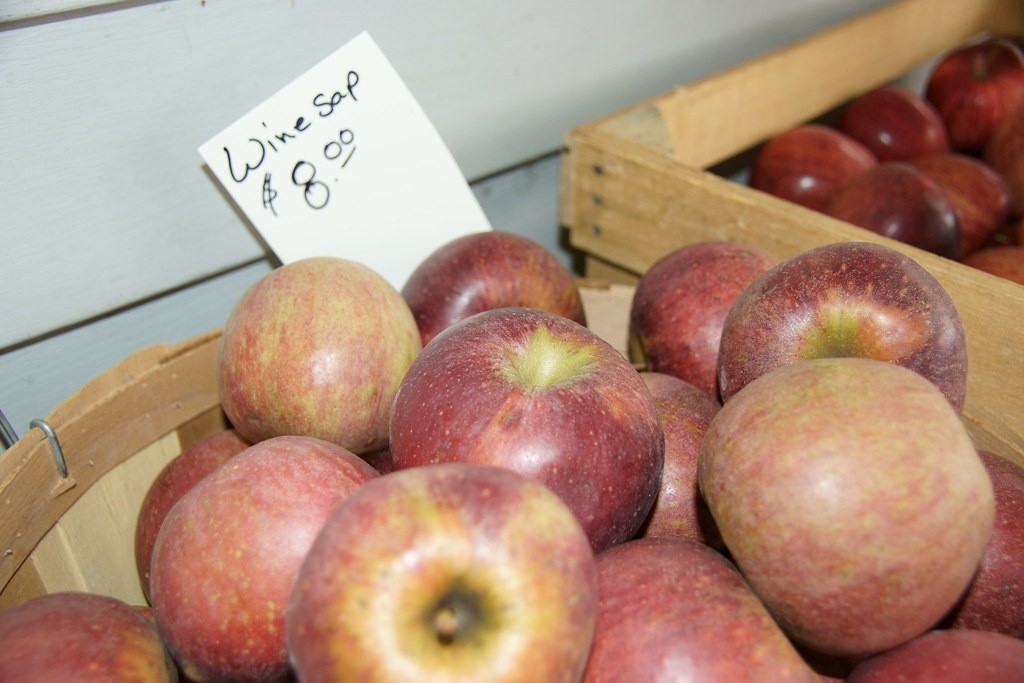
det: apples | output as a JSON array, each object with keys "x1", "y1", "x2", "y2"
[
  {"x1": 0, "y1": 230, "x2": 1024, "y2": 683},
  {"x1": 740, "y1": 28, "x2": 1024, "y2": 286}
]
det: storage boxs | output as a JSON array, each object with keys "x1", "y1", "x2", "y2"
[{"x1": 551, "y1": 1, "x2": 1024, "y2": 474}]
